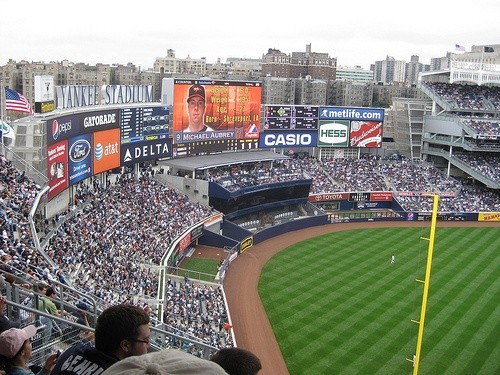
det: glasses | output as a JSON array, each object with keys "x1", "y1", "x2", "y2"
[{"x1": 124, "y1": 337, "x2": 150, "y2": 343}]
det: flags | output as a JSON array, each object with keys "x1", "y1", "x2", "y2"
[
  {"x1": 455, "y1": 44, "x2": 465, "y2": 51},
  {"x1": 5, "y1": 87, "x2": 33, "y2": 114}
]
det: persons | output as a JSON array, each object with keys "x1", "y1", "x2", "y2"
[
  {"x1": 182, "y1": 85, "x2": 214, "y2": 132},
  {"x1": 0, "y1": 82, "x2": 500, "y2": 375}
]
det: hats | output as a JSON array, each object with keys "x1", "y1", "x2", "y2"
[
  {"x1": 100, "y1": 348, "x2": 231, "y2": 375},
  {"x1": 0, "y1": 324, "x2": 36, "y2": 359},
  {"x1": 186, "y1": 84, "x2": 205, "y2": 102}
]
